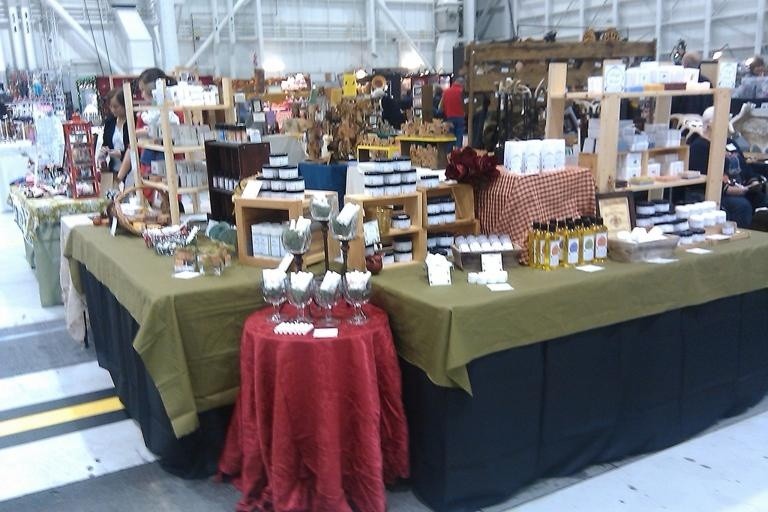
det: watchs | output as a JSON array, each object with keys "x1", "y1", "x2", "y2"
[
  {"x1": 723, "y1": 184, "x2": 729, "y2": 193},
  {"x1": 114, "y1": 177, "x2": 122, "y2": 185}
]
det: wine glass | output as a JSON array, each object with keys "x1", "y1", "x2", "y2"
[
  {"x1": 259, "y1": 265, "x2": 288, "y2": 327},
  {"x1": 342, "y1": 270, "x2": 370, "y2": 325},
  {"x1": 314, "y1": 273, "x2": 341, "y2": 327},
  {"x1": 289, "y1": 272, "x2": 311, "y2": 331}
]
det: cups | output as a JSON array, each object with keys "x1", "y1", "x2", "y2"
[
  {"x1": 309, "y1": 195, "x2": 333, "y2": 220},
  {"x1": 333, "y1": 211, "x2": 355, "y2": 242},
  {"x1": 364, "y1": 204, "x2": 391, "y2": 234},
  {"x1": 282, "y1": 220, "x2": 312, "y2": 255}
]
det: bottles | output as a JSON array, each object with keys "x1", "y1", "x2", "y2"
[
  {"x1": 213, "y1": 123, "x2": 249, "y2": 146},
  {"x1": 212, "y1": 170, "x2": 240, "y2": 192},
  {"x1": 528, "y1": 215, "x2": 609, "y2": 271}
]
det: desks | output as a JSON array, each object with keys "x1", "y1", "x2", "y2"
[
  {"x1": 5, "y1": 174, "x2": 273, "y2": 479},
  {"x1": 219, "y1": 301, "x2": 411, "y2": 512},
  {"x1": 373, "y1": 224, "x2": 768, "y2": 511}
]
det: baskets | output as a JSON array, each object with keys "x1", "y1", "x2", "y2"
[{"x1": 107, "y1": 184, "x2": 172, "y2": 234}]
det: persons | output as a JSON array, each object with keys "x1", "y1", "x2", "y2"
[
  {"x1": 472, "y1": 92, "x2": 490, "y2": 150},
  {"x1": 681, "y1": 51, "x2": 713, "y2": 88},
  {"x1": 113, "y1": 67, "x2": 185, "y2": 213},
  {"x1": 381, "y1": 95, "x2": 405, "y2": 130},
  {"x1": 401, "y1": 89, "x2": 412, "y2": 108},
  {"x1": 438, "y1": 76, "x2": 467, "y2": 154},
  {"x1": 741, "y1": 56, "x2": 764, "y2": 85},
  {"x1": 97, "y1": 87, "x2": 137, "y2": 203},
  {"x1": 687, "y1": 105, "x2": 768, "y2": 228},
  {"x1": 433, "y1": 86, "x2": 443, "y2": 118}
]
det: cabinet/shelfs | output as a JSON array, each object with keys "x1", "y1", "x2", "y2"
[{"x1": 119, "y1": 33, "x2": 732, "y2": 273}]
